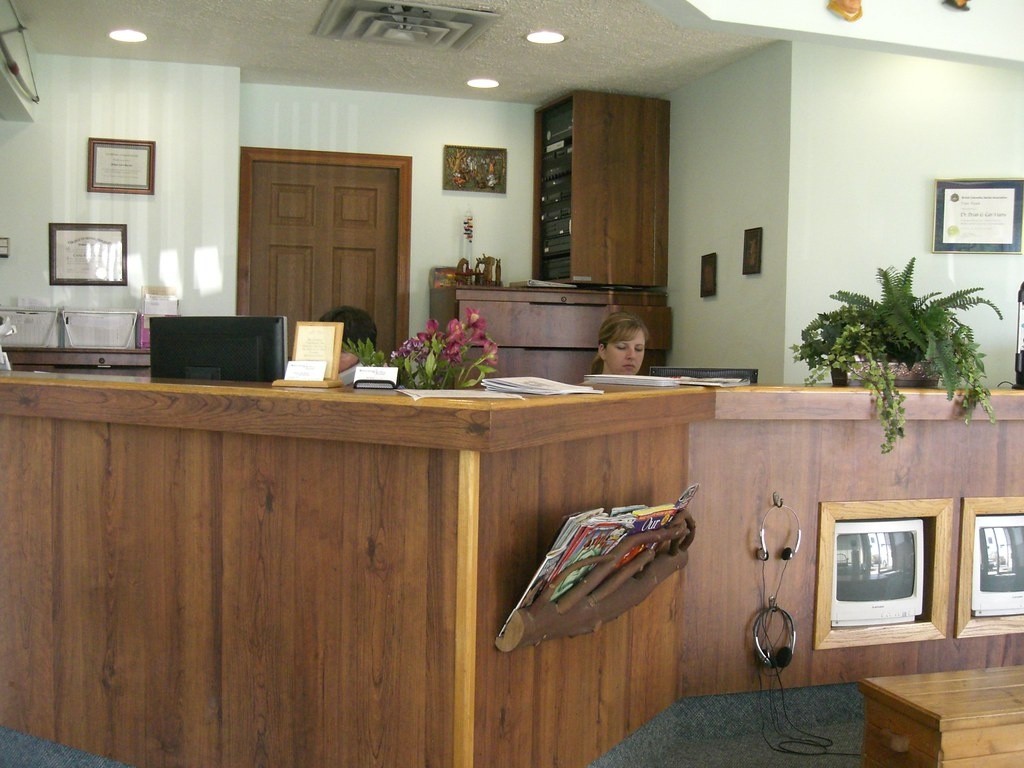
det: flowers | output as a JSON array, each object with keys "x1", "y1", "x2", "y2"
[{"x1": 341, "y1": 309, "x2": 501, "y2": 391}]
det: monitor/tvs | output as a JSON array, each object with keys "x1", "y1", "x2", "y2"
[
  {"x1": 648, "y1": 366, "x2": 759, "y2": 383},
  {"x1": 149, "y1": 316, "x2": 288, "y2": 383}
]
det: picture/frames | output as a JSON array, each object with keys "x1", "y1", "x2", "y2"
[
  {"x1": 742, "y1": 227, "x2": 763, "y2": 274},
  {"x1": 700, "y1": 253, "x2": 716, "y2": 297},
  {"x1": 49, "y1": 223, "x2": 128, "y2": 286},
  {"x1": 88, "y1": 138, "x2": 155, "y2": 196}
]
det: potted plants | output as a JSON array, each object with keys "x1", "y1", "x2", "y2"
[{"x1": 790, "y1": 254, "x2": 1003, "y2": 451}]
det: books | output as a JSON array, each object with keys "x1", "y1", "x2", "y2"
[
  {"x1": 510, "y1": 280, "x2": 662, "y2": 292},
  {"x1": 480, "y1": 376, "x2": 604, "y2": 396},
  {"x1": 584, "y1": 374, "x2": 751, "y2": 387},
  {"x1": 499, "y1": 504, "x2": 678, "y2": 637}
]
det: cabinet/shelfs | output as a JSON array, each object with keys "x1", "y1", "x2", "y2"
[{"x1": 532, "y1": 88, "x2": 670, "y2": 286}]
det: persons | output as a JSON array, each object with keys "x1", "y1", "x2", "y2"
[
  {"x1": 318, "y1": 306, "x2": 406, "y2": 389},
  {"x1": 589, "y1": 310, "x2": 650, "y2": 375}
]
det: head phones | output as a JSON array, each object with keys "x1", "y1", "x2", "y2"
[
  {"x1": 753, "y1": 607, "x2": 797, "y2": 669},
  {"x1": 757, "y1": 505, "x2": 801, "y2": 560}
]
